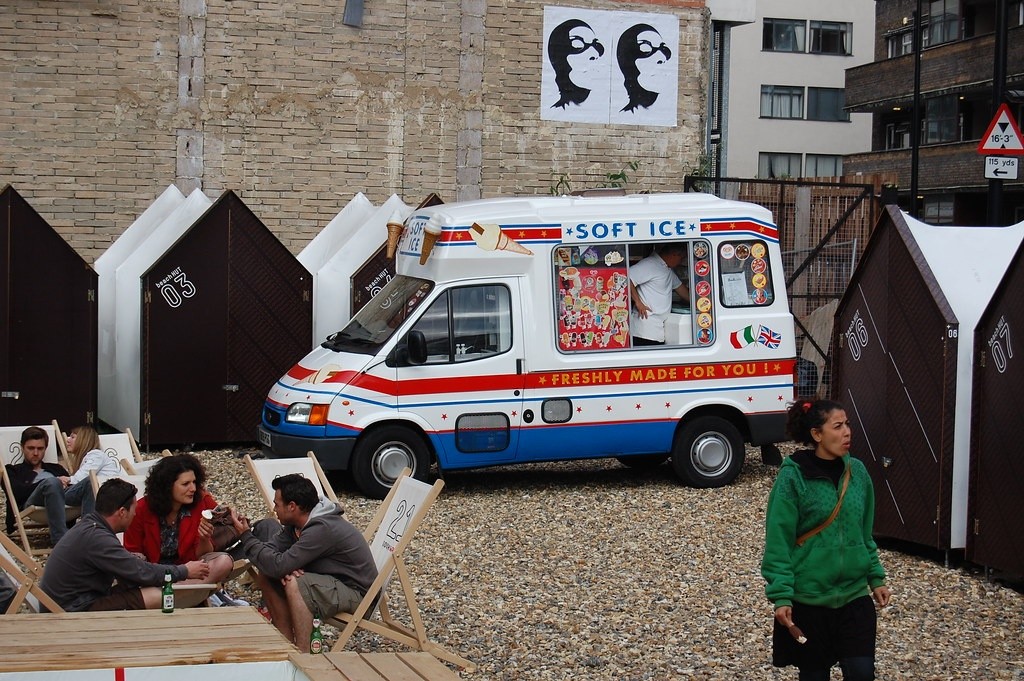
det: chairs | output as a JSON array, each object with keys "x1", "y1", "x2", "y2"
[
  {"x1": 0, "y1": 420, "x2": 350, "y2": 614},
  {"x1": 319, "y1": 467, "x2": 478, "y2": 652}
]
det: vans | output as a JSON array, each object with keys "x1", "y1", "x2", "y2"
[{"x1": 252, "y1": 188, "x2": 802, "y2": 498}]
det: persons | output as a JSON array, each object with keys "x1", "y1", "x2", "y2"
[
  {"x1": 66, "y1": 425, "x2": 129, "y2": 487},
  {"x1": 629, "y1": 242, "x2": 690, "y2": 347},
  {"x1": 0, "y1": 427, "x2": 96, "y2": 544},
  {"x1": 231, "y1": 473, "x2": 382, "y2": 653},
  {"x1": 760, "y1": 400, "x2": 891, "y2": 681},
  {"x1": 38, "y1": 477, "x2": 210, "y2": 613},
  {"x1": 123, "y1": 454, "x2": 234, "y2": 584}
]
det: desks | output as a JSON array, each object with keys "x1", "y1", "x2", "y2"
[
  {"x1": 284, "y1": 652, "x2": 465, "y2": 681},
  {"x1": 0, "y1": 605, "x2": 302, "y2": 681}
]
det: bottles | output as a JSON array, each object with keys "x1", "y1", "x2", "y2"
[
  {"x1": 161, "y1": 569, "x2": 175, "y2": 613},
  {"x1": 309, "y1": 614, "x2": 322, "y2": 654}
]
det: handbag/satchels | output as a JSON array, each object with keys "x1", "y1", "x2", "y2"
[{"x1": 209, "y1": 509, "x2": 248, "y2": 551}]
